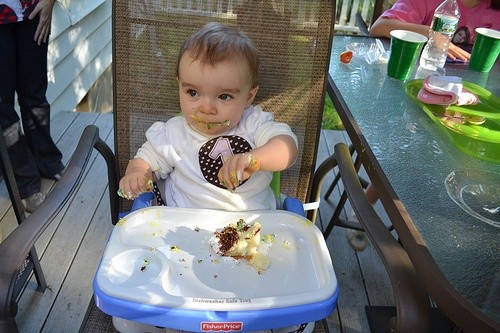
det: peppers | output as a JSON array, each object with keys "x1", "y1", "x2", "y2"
[{"x1": 340, "y1": 51, "x2": 352, "y2": 63}]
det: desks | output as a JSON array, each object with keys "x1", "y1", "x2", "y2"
[{"x1": 325, "y1": 34, "x2": 499, "y2": 332}]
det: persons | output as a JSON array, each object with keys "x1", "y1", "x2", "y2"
[
  {"x1": 0, "y1": 0, "x2": 65, "y2": 211},
  {"x1": 119, "y1": 23, "x2": 299, "y2": 210},
  {"x1": 347, "y1": 0, "x2": 500, "y2": 250}
]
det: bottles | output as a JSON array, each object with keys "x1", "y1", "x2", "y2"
[{"x1": 420, "y1": 0, "x2": 460, "y2": 72}]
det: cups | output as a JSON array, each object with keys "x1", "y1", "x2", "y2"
[
  {"x1": 387, "y1": 30, "x2": 428, "y2": 81},
  {"x1": 469, "y1": 27, "x2": 500, "y2": 73}
]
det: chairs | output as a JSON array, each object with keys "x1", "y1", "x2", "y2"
[
  {"x1": 0, "y1": 1, "x2": 432, "y2": 333},
  {"x1": 322, "y1": 0, "x2": 402, "y2": 240},
  {"x1": 90, "y1": 172, "x2": 339, "y2": 333}
]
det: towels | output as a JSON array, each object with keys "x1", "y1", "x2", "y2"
[{"x1": 417, "y1": 73, "x2": 464, "y2": 106}]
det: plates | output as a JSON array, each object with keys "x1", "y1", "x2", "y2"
[
  {"x1": 403, "y1": 78, "x2": 500, "y2": 164},
  {"x1": 445, "y1": 167, "x2": 500, "y2": 228}
]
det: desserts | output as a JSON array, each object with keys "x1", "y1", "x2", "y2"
[{"x1": 209, "y1": 219, "x2": 273, "y2": 273}]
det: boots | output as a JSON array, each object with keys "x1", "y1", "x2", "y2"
[
  {"x1": 0, "y1": 109, "x2": 42, "y2": 199},
  {"x1": 20, "y1": 104, "x2": 65, "y2": 178}
]
